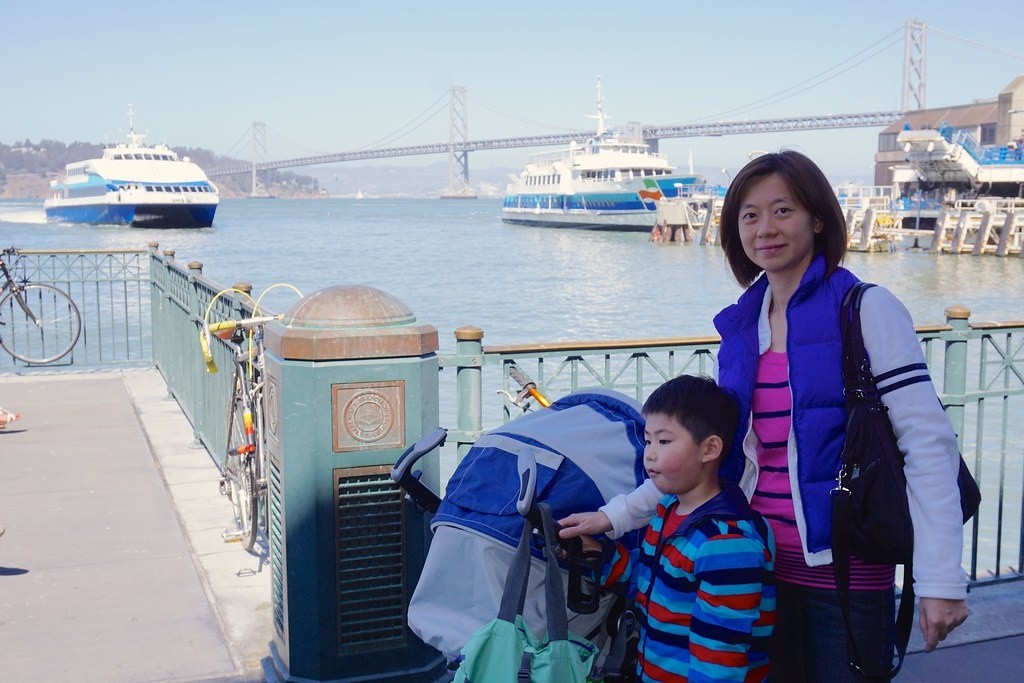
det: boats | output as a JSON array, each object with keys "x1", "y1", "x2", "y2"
[
  {"x1": 43, "y1": 103, "x2": 222, "y2": 230},
  {"x1": 499, "y1": 71, "x2": 710, "y2": 230}
]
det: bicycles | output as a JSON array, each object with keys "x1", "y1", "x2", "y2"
[
  {"x1": 197, "y1": 283, "x2": 304, "y2": 556},
  {"x1": 0, "y1": 244, "x2": 84, "y2": 366}
]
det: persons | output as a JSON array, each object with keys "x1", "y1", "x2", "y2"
[
  {"x1": 556, "y1": 150, "x2": 972, "y2": 683},
  {"x1": 622, "y1": 375, "x2": 777, "y2": 683}
]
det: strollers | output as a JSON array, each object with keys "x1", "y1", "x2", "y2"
[{"x1": 388, "y1": 386, "x2": 648, "y2": 683}]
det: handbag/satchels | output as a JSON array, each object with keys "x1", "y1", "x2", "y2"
[
  {"x1": 830, "y1": 283, "x2": 982, "y2": 683},
  {"x1": 450, "y1": 503, "x2": 605, "y2": 683}
]
